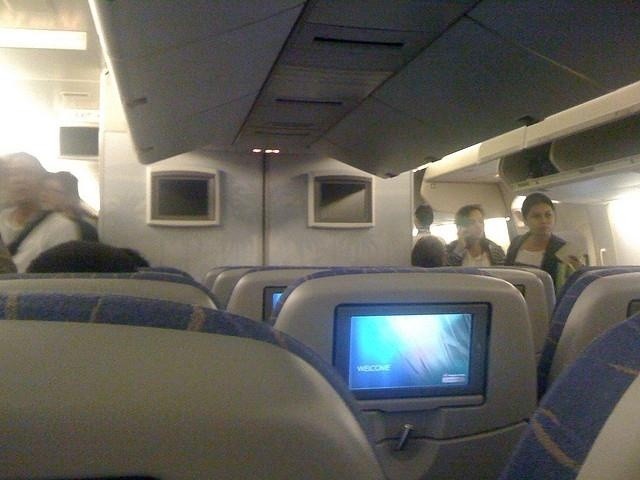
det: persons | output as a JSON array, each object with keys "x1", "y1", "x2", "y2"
[
  {"x1": 0, "y1": 152, "x2": 149, "y2": 274},
  {"x1": 414, "y1": 204, "x2": 434, "y2": 246},
  {"x1": 445, "y1": 203, "x2": 506, "y2": 265},
  {"x1": 506, "y1": 191, "x2": 570, "y2": 297},
  {"x1": 410, "y1": 233, "x2": 447, "y2": 267}
]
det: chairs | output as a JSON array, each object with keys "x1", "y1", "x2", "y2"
[{"x1": 1, "y1": 265, "x2": 640, "y2": 480}]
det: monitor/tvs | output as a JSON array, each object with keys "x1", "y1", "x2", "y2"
[
  {"x1": 333, "y1": 302, "x2": 490, "y2": 412},
  {"x1": 307, "y1": 169, "x2": 375, "y2": 229},
  {"x1": 57, "y1": 121, "x2": 101, "y2": 162},
  {"x1": 264, "y1": 287, "x2": 286, "y2": 319},
  {"x1": 145, "y1": 164, "x2": 221, "y2": 227}
]
